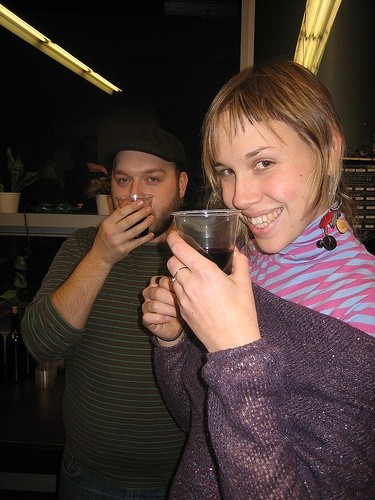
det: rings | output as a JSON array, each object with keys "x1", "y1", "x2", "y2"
[
  {"x1": 170, "y1": 266, "x2": 188, "y2": 283},
  {"x1": 154, "y1": 273, "x2": 162, "y2": 286}
]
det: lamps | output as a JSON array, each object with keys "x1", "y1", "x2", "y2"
[
  {"x1": 0, "y1": 3, "x2": 124, "y2": 96},
  {"x1": 294, "y1": 0, "x2": 343, "y2": 78}
]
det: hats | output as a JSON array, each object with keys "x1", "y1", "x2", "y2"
[{"x1": 112, "y1": 127, "x2": 188, "y2": 162}]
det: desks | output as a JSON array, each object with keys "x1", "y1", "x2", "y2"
[{"x1": 0, "y1": 379, "x2": 65, "y2": 500}]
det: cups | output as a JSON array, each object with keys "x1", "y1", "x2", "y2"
[
  {"x1": 114, "y1": 195, "x2": 153, "y2": 243},
  {"x1": 171, "y1": 209, "x2": 237, "y2": 278}
]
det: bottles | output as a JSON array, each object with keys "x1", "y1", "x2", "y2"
[{"x1": 5, "y1": 306, "x2": 27, "y2": 382}]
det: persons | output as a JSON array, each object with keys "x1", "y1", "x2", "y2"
[
  {"x1": 136, "y1": 58, "x2": 375, "y2": 500},
  {"x1": 21, "y1": 127, "x2": 210, "y2": 500}
]
